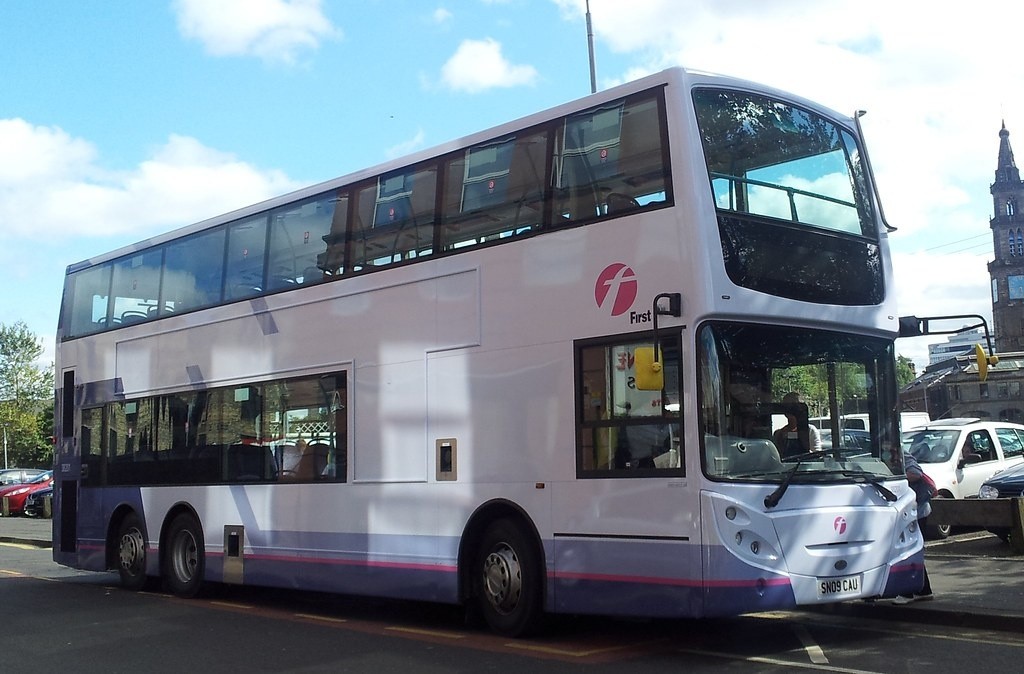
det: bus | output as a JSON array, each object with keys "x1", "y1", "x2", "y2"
[{"x1": 51, "y1": 72, "x2": 1001, "y2": 639}]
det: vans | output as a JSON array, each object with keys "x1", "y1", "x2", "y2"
[{"x1": 806, "y1": 411, "x2": 930, "y2": 457}]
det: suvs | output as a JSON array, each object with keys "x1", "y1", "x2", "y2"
[{"x1": 892, "y1": 417, "x2": 1023, "y2": 538}]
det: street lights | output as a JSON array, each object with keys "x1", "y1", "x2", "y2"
[{"x1": 0, "y1": 422, "x2": 11, "y2": 470}]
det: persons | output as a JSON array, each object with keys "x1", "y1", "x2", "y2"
[
  {"x1": 893, "y1": 448, "x2": 933, "y2": 605},
  {"x1": 962, "y1": 441, "x2": 974, "y2": 458},
  {"x1": 774, "y1": 392, "x2": 821, "y2": 453},
  {"x1": 626, "y1": 391, "x2": 681, "y2": 467},
  {"x1": 296, "y1": 440, "x2": 306, "y2": 451}
]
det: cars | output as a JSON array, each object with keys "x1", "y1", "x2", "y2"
[
  {"x1": 0, "y1": 469, "x2": 46, "y2": 488},
  {"x1": 981, "y1": 461, "x2": 1024, "y2": 540},
  {"x1": 24, "y1": 486, "x2": 54, "y2": 519},
  {"x1": 0, "y1": 470, "x2": 54, "y2": 513}
]
